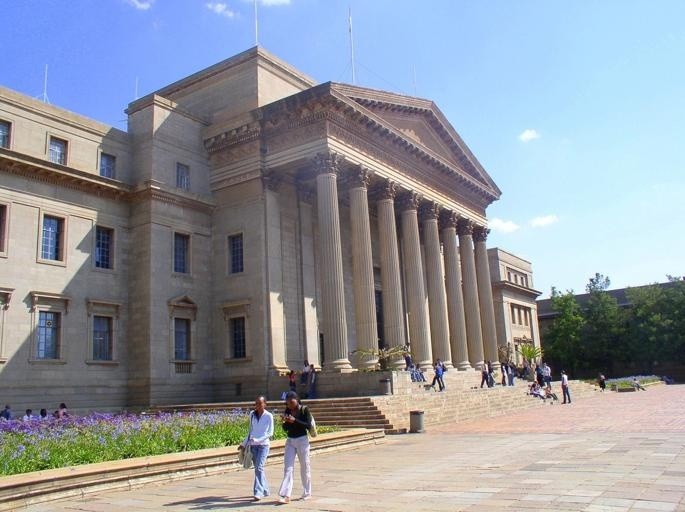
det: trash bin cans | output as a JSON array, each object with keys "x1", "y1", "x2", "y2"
[
  {"x1": 379, "y1": 379, "x2": 392, "y2": 395},
  {"x1": 611, "y1": 385, "x2": 616, "y2": 390},
  {"x1": 409, "y1": 411, "x2": 425, "y2": 433}
]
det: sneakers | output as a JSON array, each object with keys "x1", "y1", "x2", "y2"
[{"x1": 250, "y1": 494, "x2": 312, "y2": 505}]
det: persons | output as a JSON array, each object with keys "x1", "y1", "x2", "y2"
[
  {"x1": 633, "y1": 378, "x2": 646, "y2": 391},
  {"x1": 307, "y1": 364, "x2": 316, "y2": 398},
  {"x1": 435, "y1": 358, "x2": 445, "y2": 390},
  {"x1": 560, "y1": 370, "x2": 572, "y2": 404},
  {"x1": 275, "y1": 391, "x2": 313, "y2": 504},
  {"x1": 402, "y1": 342, "x2": 412, "y2": 370},
  {"x1": 406, "y1": 364, "x2": 418, "y2": 382},
  {"x1": 479, "y1": 360, "x2": 559, "y2": 401},
  {"x1": 414, "y1": 363, "x2": 426, "y2": 381},
  {"x1": 299, "y1": 360, "x2": 310, "y2": 387},
  {"x1": 287, "y1": 369, "x2": 295, "y2": 390},
  {"x1": 599, "y1": 372, "x2": 606, "y2": 392},
  {"x1": 237, "y1": 393, "x2": 272, "y2": 501},
  {"x1": 431, "y1": 362, "x2": 443, "y2": 392}
]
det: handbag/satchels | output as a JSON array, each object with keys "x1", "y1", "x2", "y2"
[
  {"x1": 302, "y1": 405, "x2": 317, "y2": 438},
  {"x1": 239, "y1": 443, "x2": 253, "y2": 469}
]
